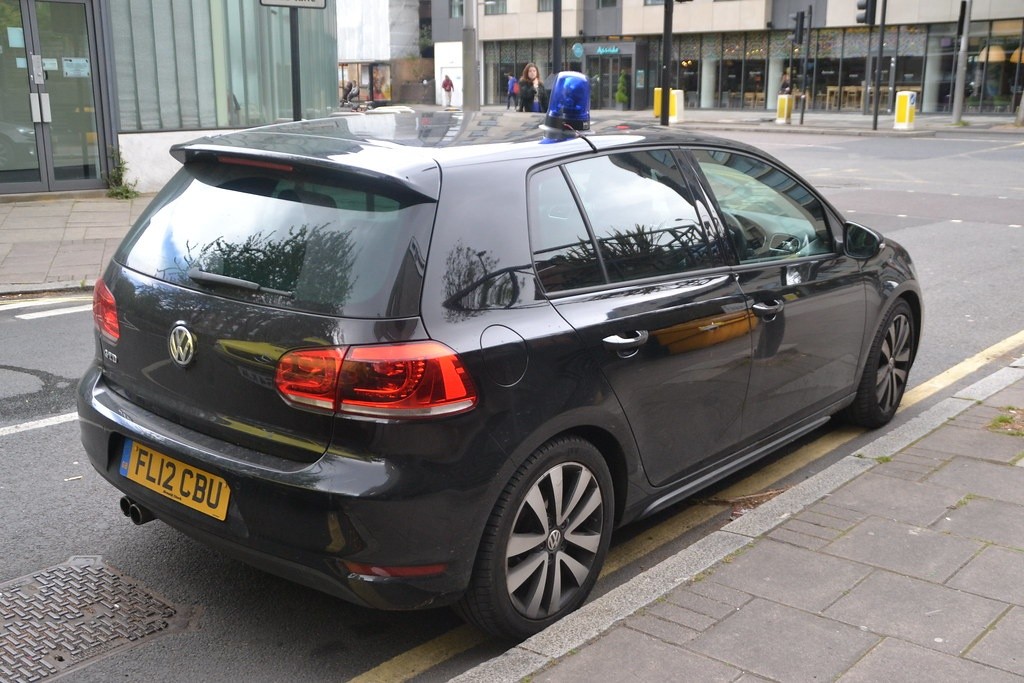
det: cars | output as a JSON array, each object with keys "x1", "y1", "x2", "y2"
[{"x1": 77, "y1": 70, "x2": 925, "y2": 640}]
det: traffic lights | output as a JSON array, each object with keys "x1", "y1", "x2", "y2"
[
  {"x1": 788, "y1": 11, "x2": 804, "y2": 44},
  {"x1": 856, "y1": 0, "x2": 877, "y2": 24}
]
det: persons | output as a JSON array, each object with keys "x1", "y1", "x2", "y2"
[
  {"x1": 442, "y1": 75, "x2": 455, "y2": 106},
  {"x1": 348, "y1": 81, "x2": 360, "y2": 102},
  {"x1": 373, "y1": 78, "x2": 385, "y2": 99},
  {"x1": 340, "y1": 82, "x2": 351, "y2": 103},
  {"x1": 517, "y1": 63, "x2": 548, "y2": 112},
  {"x1": 507, "y1": 73, "x2": 518, "y2": 110}
]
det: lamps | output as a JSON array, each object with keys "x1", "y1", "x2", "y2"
[
  {"x1": 1008, "y1": 39, "x2": 1024, "y2": 64},
  {"x1": 977, "y1": 38, "x2": 1007, "y2": 63}
]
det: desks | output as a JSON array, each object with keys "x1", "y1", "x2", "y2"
[
  {"x1": 827, "y1": 87, "x2": 922, "y2": 110},
  {"x1": 817, "y1": 94, "x2": 826, "y2": 109}
]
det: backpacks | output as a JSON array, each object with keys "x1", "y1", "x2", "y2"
[{"x1": 512, "y1": 79, "x2": 519, "y2": 94}]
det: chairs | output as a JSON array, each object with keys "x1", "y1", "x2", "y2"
[
  {"x1": 687, "y1": 91, "x2": 765, "y2": 110},
  {"x1": 938, "y1": 96, "x2": 949, "y2": 112},
  {"x1": 825, "y1": 85, "x2": 922, "y2": 111}
]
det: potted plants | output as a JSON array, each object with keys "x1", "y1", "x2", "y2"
[{"x1": 615, "y1": 69, "x2": 630, "y2": 110}]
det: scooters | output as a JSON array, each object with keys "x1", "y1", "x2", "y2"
[{"x1": 351, "y1": 101, "x2": 393, "y2": 112}]
